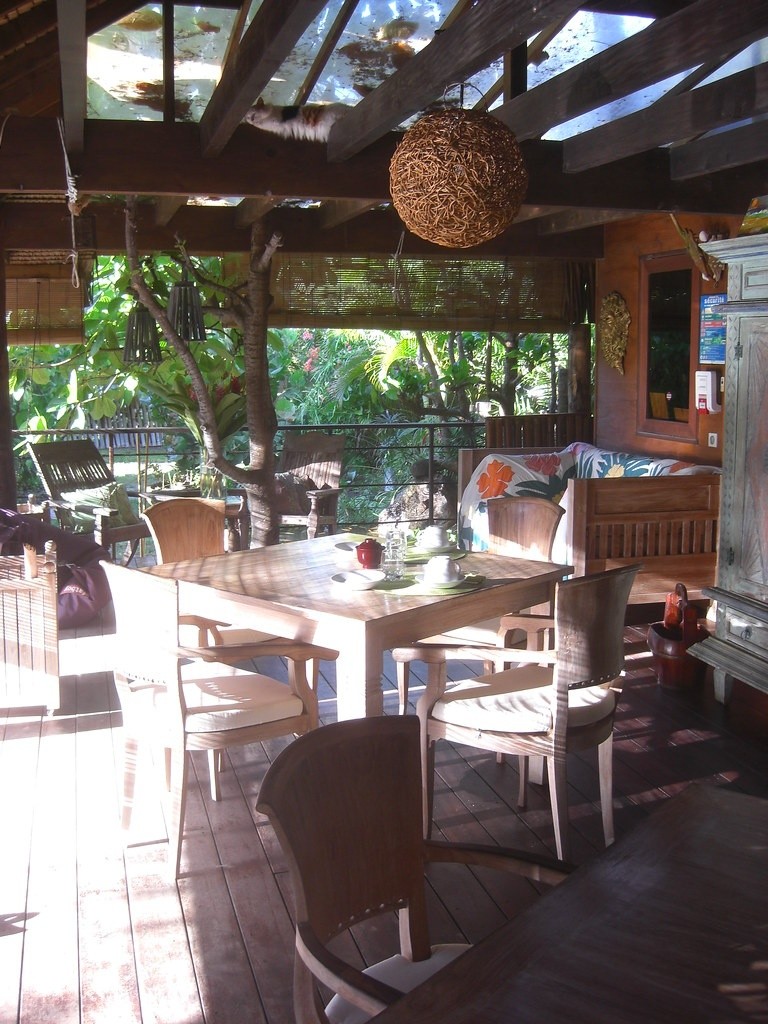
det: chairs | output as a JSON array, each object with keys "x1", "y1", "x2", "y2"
[
  {"x1": 139, "y1": 498, "x2": 226, "y2": 564},
  {"x1": 99, "y1": 559, "x2": 309, "y2": 877},
  {"x1": 256, "y1": 716, "x2": 578, "y2": 1024},
  {"x1": 417, "y1": 563, "x2": 645, "y2": 861},
  {"x1": 25, "y1": 439, "x2": 152, "y2": 550},
  {"x1": 226, "y1": 432, "x2": 346, "y2": 551},
  {"x1": 483, "y1": 497, "x2": 566, "y2": 675}
]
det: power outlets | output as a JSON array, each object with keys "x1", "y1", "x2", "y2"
[{"x1": 708, "y1": 433, "x2": 717, "y2": 447}]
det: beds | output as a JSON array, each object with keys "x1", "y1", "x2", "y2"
[{"x1": 457, "y1": 442, "x2": 722, "y2": 605}]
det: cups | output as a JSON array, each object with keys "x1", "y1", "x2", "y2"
[
  {"x1": 356, "y1": 538, "x2": 386, "y2": 568},
  {"x1": 334, "y1": 542, "x2": 359, "y2": 569},
  {"x1": 386, "y1": 530, "x2": 407, "y2": 558},
  {"x1": 424, "y1": 556, "x2": 460, "y2": 581},
  {"x1": 380, "y1": 549, "x2": 405, "y2": 582},
  {"x1": 417, "y1": 526, "x2": 448, "y2": 547}
]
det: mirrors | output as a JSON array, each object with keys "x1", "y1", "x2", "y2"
[{"x1": 636, "y1": 248, "x2": 701, "y2": 445}]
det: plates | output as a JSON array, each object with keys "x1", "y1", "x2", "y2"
[
  {"x1": 415, "y1": 575, "x2": 466, "y2": 588},
  {"x1": 331, "y1": 570, "x2": 386, "y2": 590},
  {"x1": 415, "y1": 544, "x2": 458, "y2": 552}
]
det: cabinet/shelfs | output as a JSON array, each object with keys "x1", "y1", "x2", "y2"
[{"x1": 685, "y1": 233, "x2": 768, "y2": 704}]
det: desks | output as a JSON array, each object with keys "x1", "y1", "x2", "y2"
[
  {"x1": 365, "y1": 780, "x2": 768, "y2": 1024},
  {"x1": 137, "y1": 534, "x2": 575, "y2": 722}
]
